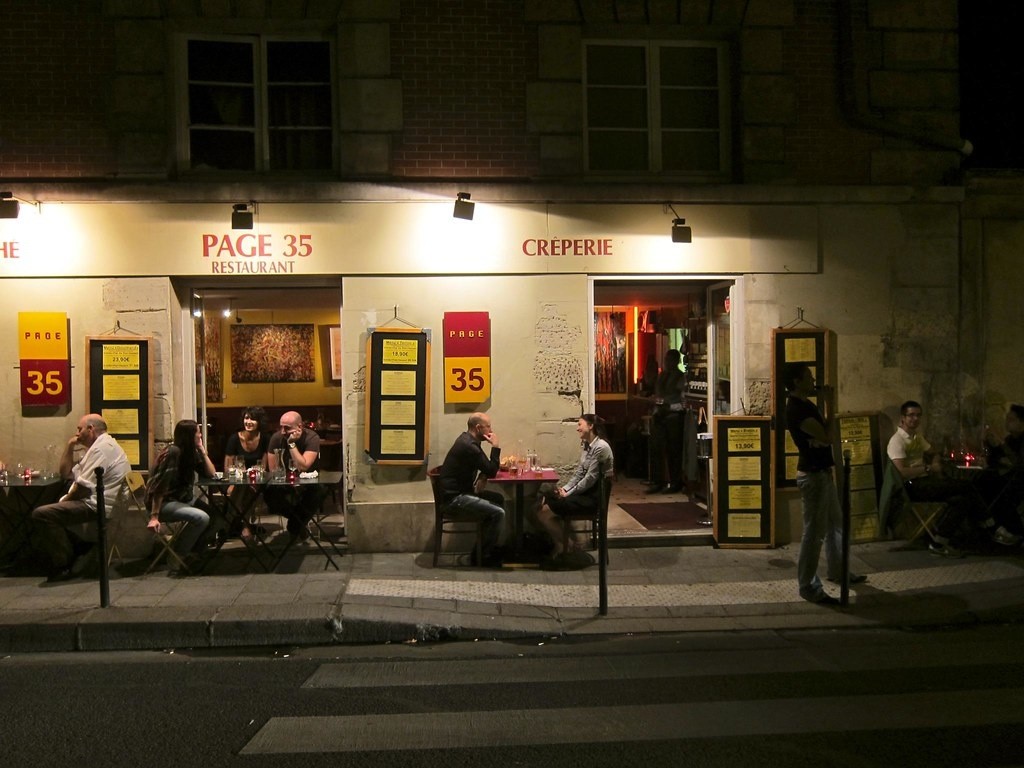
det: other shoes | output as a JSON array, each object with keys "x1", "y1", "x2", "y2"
[
  {"x1": 471, "y1": 547, "x2": 501, "y2": 569},
  {"x1": 818, "y1": 596, "x2": 840, "y2": 605},
  {"x1": 849, "y1": 573, "x2": 868, "y2": 583},
  {"x1": 47, "y1": 566, "x2": 74, "y2": 581},
  {"x1": 2, "y1": 565, "x2": 34, "y2": 577},
  {"x1": 537, "y1": 545, "x2": 594, "y2": 572}
]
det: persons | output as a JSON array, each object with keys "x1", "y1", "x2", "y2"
[
  {"x1": 31, "y1": 413, "x2": 133, "y2": 582},
  {"x1": 265, "y1": 410, "x2": 322, "y2": 545},
  {"x1": 530, "y1": 414, "x2": 613, "y2": 564},
  {"x1": 647, "y1": 350, "x2": 685, "y2": 494},
  {"x1": 886, "y1": 400, "x2": 1024, "y2": 557},
  {"x1": 224, "y1": 406, "x2": 271, "y2": 545},
  {"x1": 782, "y1": 363, "x2": 866, "y2": 605},
  {"x1": 143, "y1": 420, "x2": 216, "y2": 573},
  {"x1": 437, "y1": 412, "x2": 505, "y2": 568}
]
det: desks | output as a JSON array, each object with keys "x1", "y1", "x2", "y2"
[
  {"x1": 955, "y1": 466, "x2": 1021, "y2": 556},
  {"x1": 320, "y1": 440, "x2": 342, "y2": 503},
  {"x1": 194, "y1": 471, "x2": 343, "y2": 577},
  {"x1": 0, "y1": 476, "x2": 61, "y2": 578},
  {"x1": 487, "y1": 466, "x2": 560, "y2": 568}
]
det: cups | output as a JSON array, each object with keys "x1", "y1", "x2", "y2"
[
  {"x1": 214, "y1": 459, "x2": 298, "y2": 481},
  {"x1": 0, "y1": 463, "x2": 49, "y2": 482},
  {"x1": 509, "y1": 456, "x2": 542, "y2": 476}
]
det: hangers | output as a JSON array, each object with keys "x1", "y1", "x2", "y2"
[{"x1": 698, "y1": 400, "x2": 708, "y2": 425}]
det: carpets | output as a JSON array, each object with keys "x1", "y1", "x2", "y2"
[{"x1": 616, "y1": 502, "x2": 712, "y2": 530}]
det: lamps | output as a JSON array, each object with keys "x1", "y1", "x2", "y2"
[
  {"x1": 453, "y1": 192, "x2": 475, "y2": 221},
  {"x1": 232, "y1": 201, "x2": 258, "y2": 229},
  {"x1": 668, "y1": 204, "x2": 692, "y2": 243},
  {"x1": 236, "y1": 309, "x2": 242, "y2": 324},
  {"x1": 0, "y1": 191, "x2": 40, "y2": 218}
]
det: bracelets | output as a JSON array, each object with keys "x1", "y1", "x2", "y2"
[
  {"x1": 288, "y1": 443, "x2": 296, "y2": 448},
  {"x1": 151, "y1": 512, "x2": 159, "y2": 515}
]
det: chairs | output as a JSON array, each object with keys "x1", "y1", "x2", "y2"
[
  {"x1": 888, "y1": 458, "x2": 953, "y2": 556},
  {"x1": 67, "y1": 471, "x2": 193, "y2": 579},
  {"x1": 428, "y1": 465, "x2": 482, "y2": 569},
  {"x1": 562, "y1": 472, "x2": 614, "y2": 567}
]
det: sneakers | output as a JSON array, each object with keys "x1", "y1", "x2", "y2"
[
  {"x1": 928, "y1": 539, "x2": 966, "y2": 556},
  {"x1": 992, "y1": 526, "x2": 1021, "y2": 546}
]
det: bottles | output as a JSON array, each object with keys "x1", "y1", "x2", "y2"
[
  {"x1": 316, "y1": 407, "x2": 326, "y2": 436},
  {"x1": 524, "y1": 449, "x2": 538, "y2": 472},
  {"x1": 688, "y1": 324, "x2": 707, "y2": 394},
  {"x1": 43, "y1": 444, "x2": 58, "y2": 478},
  {"x1": 965, "y1": 452, "x2": 970, "y2": 467},
  {"x1": 272, "y1": 449, "x2": 286, "y2": 480}
]
